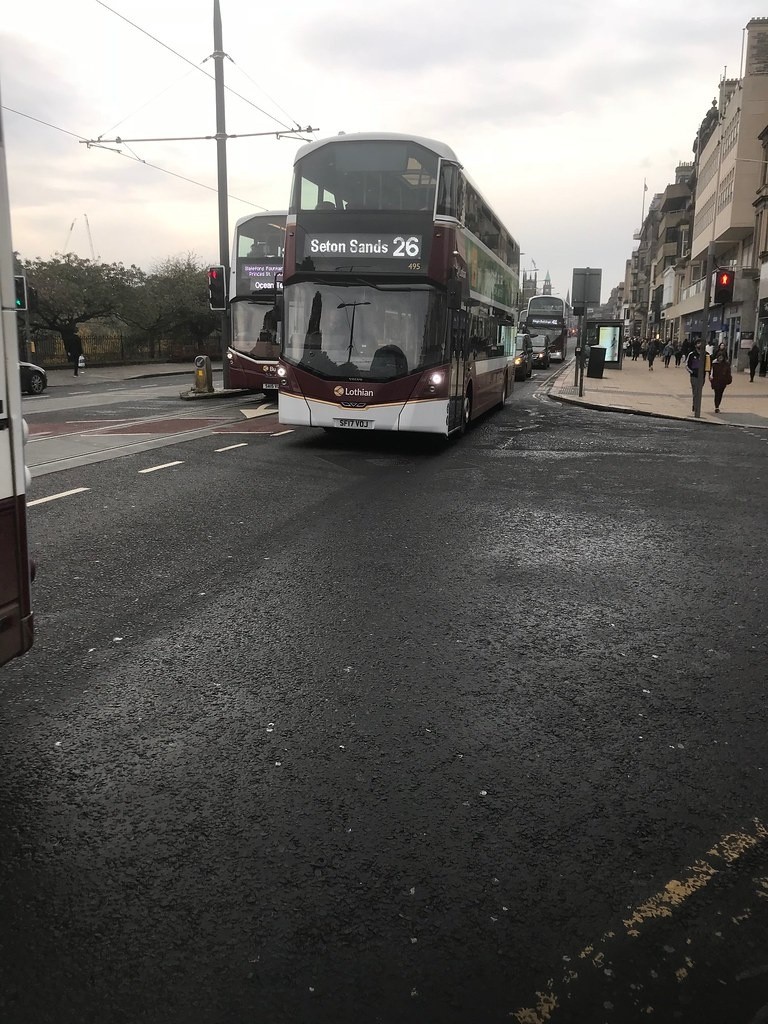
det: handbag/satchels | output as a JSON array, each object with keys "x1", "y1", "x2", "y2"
[{"x1": 78, "y1": 355, "x2": 85, "y2": 368}]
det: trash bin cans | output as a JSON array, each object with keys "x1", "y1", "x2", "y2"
[{"x1": 587, "y1": 345, "x2": 607, "y2": 379}]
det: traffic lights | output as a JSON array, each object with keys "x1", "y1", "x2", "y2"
[
  {"x1": 14, "y1": 275, "x2": 28, "y2": 311},
  {"x1": 208, "y1": 265, "x2": 227, "y2": 311},
  {"x1": 713, "y1": 271, "x2": 733, "y2": 306}
]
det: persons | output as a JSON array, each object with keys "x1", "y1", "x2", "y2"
[
  {"x1": 71, "y1": 330, "x2": 83, "y2": 378},
  {"x1": 321, "y1": 309, "x2": 412, "y2": 348},
  {"x1": 623, "y1": 336, "x2": 731, "y2": 413},
  {"x1": 747, "y1": 342, "x2": 759, "y2": 382},
  {"x1": 247, "y1": 244, "x2": 262, "y2": 258},
  {"x1": 316, "y1": 200, "x2": 335, "y2": 210}
]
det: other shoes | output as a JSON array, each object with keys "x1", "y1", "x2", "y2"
[
  {"x1": 675, "y1": 366, "x2": 677, "y2": 368},
  {"x1": 667, "y1": 366, "x2": 669, "y2": 368},
  {"x1": 750, "y1": 377, "x2": 753, "y2": 382},
  {"x1": 651, "y1": 367, "x2": 653, "y2": 370},
  {"x1": 665, "y1": 366, "x2": 666, "y2": 368},
  {"x1": 635, "y1": 358, "x2": 637, "y2": 361},
  {"x1": 678, "y1": 366, "x2": 680, "y2": 368},
  {"x1": 632, "y1": 359, "x2": 634, "y2": 360},
  {"x1": 643, "y1": 359, "x2": 646, "y2": 361},
  {"x1": 715, "y1": 409, "x2": 721, "y2": 414},
  {"x1": 649, "y1": 369, "x2": 650, "y2": 371},
  {"x1": 73, "y1": 375, "x2": 80, "y2": 378}
]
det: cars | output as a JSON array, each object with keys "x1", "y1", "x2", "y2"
[
  {"x1": 18, "y1": 358, "x2": 47, "y2": 395},
  {"x1": 513, "y1": 333, "x2": 533, "y2": 383},
  {"x1": 530, "y1": 335, "x2": 549, "y2": 369}
]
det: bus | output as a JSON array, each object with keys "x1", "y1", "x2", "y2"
[
  {"x1": 0, "y1": 108, "x2": 50, "y2": 685},
  {"x1": 226, "y1": 209, "x2": 289, "y2": 396},
  {"x1": 275, "y1": 131, "x2": 519, "y2": 440},
  {"x1": 526, "y1": 295, "x2": 568, "y2": 362}
]
